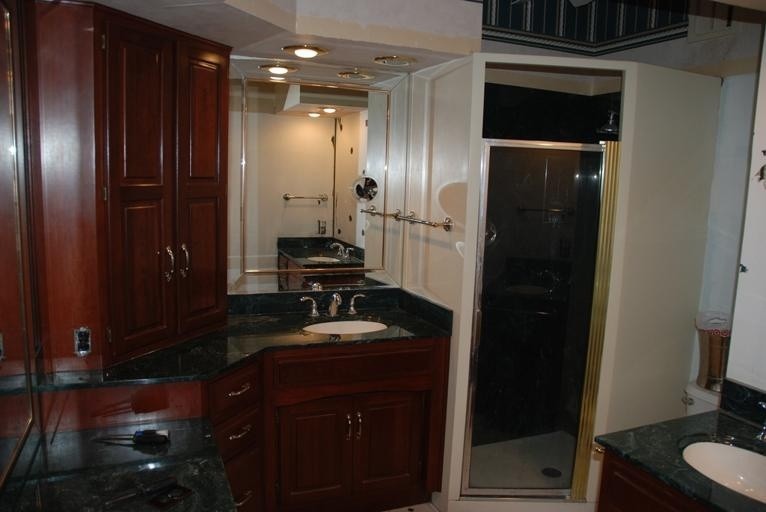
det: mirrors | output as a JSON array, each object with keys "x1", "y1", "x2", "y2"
[{"x1": 229, "y1": 74, "x2": 411, "y2": 293}]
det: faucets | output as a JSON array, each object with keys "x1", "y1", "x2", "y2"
[
  {"x1": 323, "y1": 294, "x2": 342, "y2": 317},
  {"x1": 330, "y1": 242, "x2": 346, "y2": 256}
]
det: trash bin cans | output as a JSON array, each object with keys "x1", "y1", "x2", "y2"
[{"x1": 697, "y1": 313, "x2": 732, "y2": 392}]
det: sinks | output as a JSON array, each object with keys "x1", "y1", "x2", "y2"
[
  {"x1": 506, "y1": 282, "x2": 548, "y2": 295},
  {"x1": 675, "y1": 433, "x2": 764, "y2": 508},
  {"x1": 302, "y1": 316, "x2": 389, "y2": 334},
  {"x1": 308, "y1": 256, "x2": 340, "y2": 262}
]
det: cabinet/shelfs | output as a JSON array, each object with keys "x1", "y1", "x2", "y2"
[
  {"x1": 14, "y1": 0, "x2": 231, "y2": 373},
  {"x1": 262, "y1": 341, "x2": 443, "y2": 512},
  {"x1": 278, "y1": 253, "x2": 302, "y2": 291},
  {"x1": 593, "y1": 448, "x2": 706, "y2": 512},
  {"x1": 75, "y1": 350, "x2": 266, "y2": 510}
]
